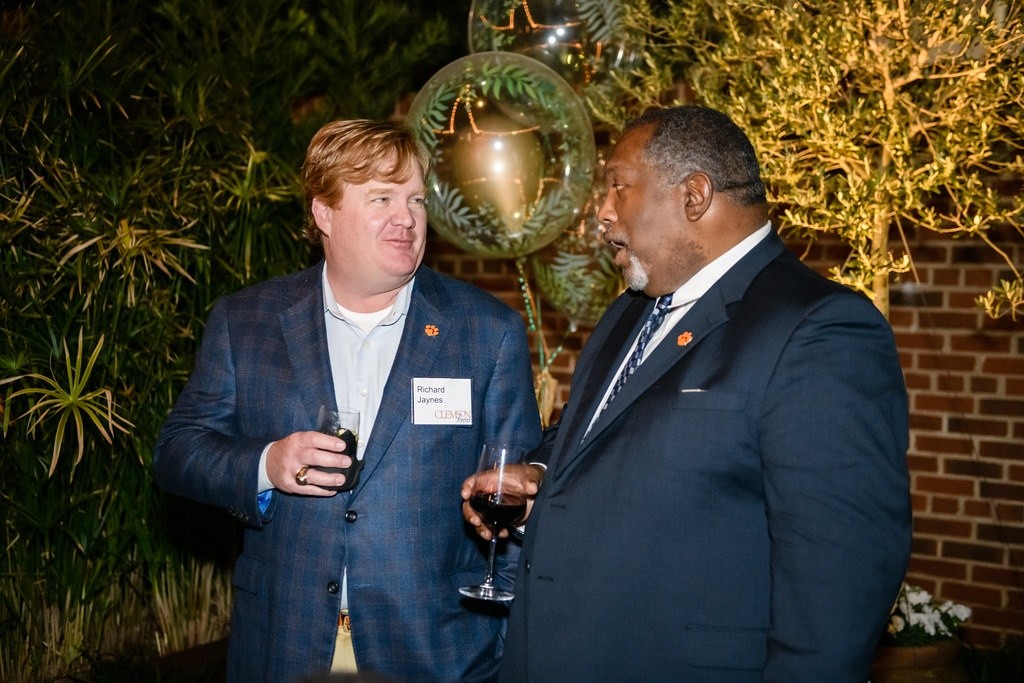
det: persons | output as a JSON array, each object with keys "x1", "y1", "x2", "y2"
[
  {"x1": 461, "y1": 106, "x2": 913, "y2": 683},
  {"x1": 148, "y1": 118, "x2": 546, "y2": 683}
]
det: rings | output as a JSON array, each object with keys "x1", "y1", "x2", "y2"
[{"x1": 295, "y1": 464, "x2": 310, "y2": 485}]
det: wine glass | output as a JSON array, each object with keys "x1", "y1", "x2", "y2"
[{"x1": 458, "y1": 441, "x2": 528, "y2": 603}]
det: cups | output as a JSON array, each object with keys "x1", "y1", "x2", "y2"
[{"x1": 310, "y1": 405, "x2": 360, "y2": 493}]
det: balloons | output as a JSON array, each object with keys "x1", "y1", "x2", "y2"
[
  {"x1": 531, "y1": 143, "x2": 631, "y2": 328},
  {"x1": 465, "y1": 1, "x2": 665, "y2": 132},
  {"x1": 398, "y1": 51, "x2": 595, "y2": 259}
]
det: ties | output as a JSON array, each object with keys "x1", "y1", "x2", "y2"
[{"x1": 581, "y1": 294, "x2": 672, "y2": 447}]
